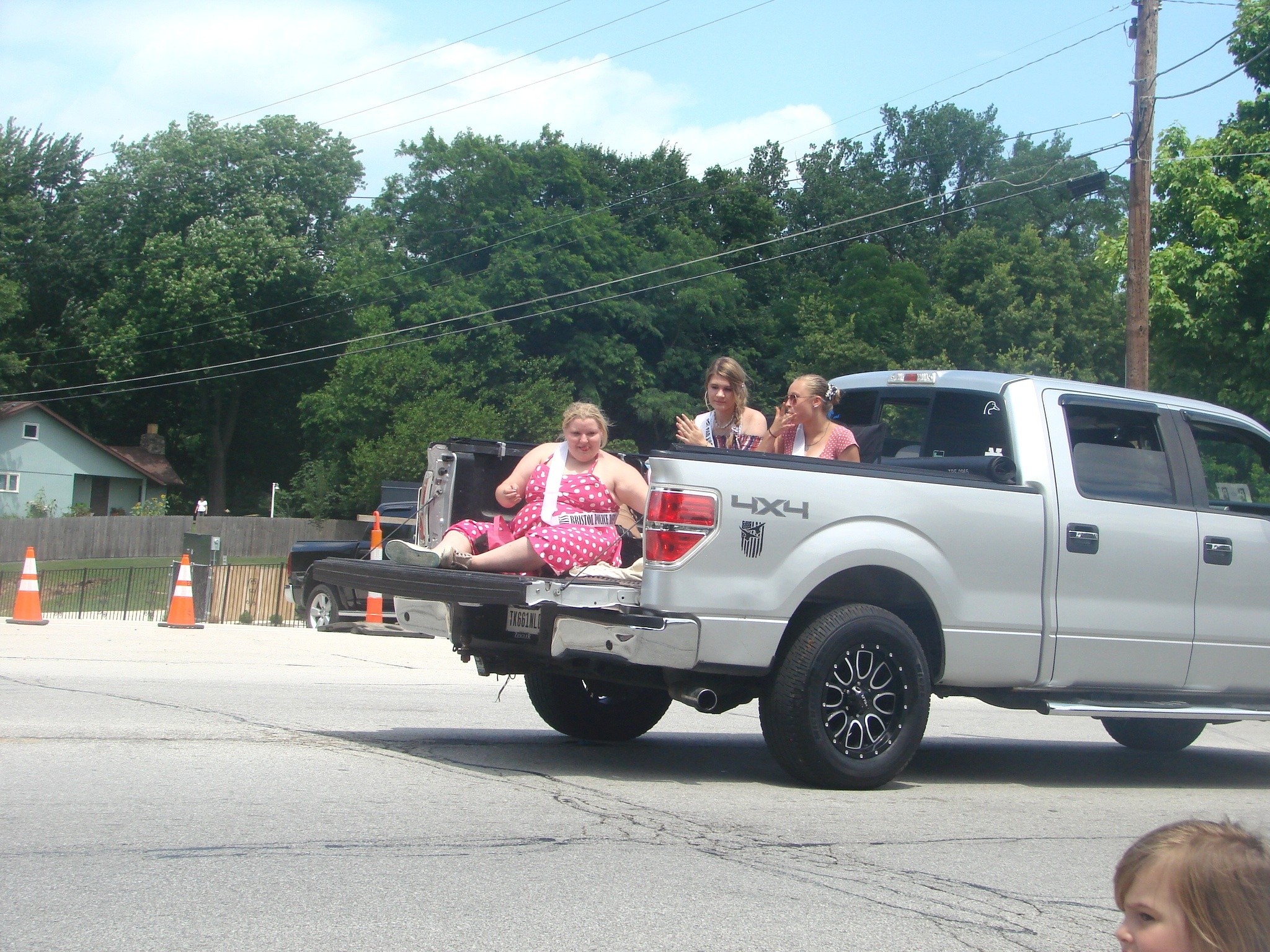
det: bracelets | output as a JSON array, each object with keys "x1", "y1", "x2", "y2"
[
  {"x1": 768, "y1": 428, "x2": 777, "y2": 439},
  {"x1": 705, "y1": 441, "x2": 709, "y2": 446}
]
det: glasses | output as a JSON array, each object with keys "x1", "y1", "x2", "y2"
[{"x1": 785, "y1": 394, "x2": 826, "y2": 405}]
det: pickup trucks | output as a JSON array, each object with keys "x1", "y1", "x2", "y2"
[
  {"x1": 310, "y1": 369, "x2": 1270, "y2": 791},
  {"x1": 284, "y1": 499, "x2": 420, "y2": 629}
]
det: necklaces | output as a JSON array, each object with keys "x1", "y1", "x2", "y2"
[
  {"x1": 712, "y1": 406, "x2": 735, "y2": 429},
  {"x1": 805, "y1": 419, "x2": 831, "y2": 451}
]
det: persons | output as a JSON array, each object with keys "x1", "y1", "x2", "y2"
[
  {"x1": 384, "y1": 402, "x2": 650, "y2": 574},
  {"x1": 754, "y1": 374, "x2": 860, "y2": 463},
  {"x1": 675, "y1": 356, "x2": 768, "y2": 451},
  {"x1": 1114, "y1": 819, "x2": 1270, "y2": 952}
]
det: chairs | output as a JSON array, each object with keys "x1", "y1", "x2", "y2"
[{"x1": 1098, "y1": 433, "x2": 1162, "y2": 498}]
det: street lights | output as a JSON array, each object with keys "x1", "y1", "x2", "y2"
[{"x1": 270, "y1": 482, "x2": 280, "y2": 518}]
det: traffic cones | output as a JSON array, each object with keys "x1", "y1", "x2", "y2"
[
  {"x1": 6, "y1": 546, "x2": 49, "y2": 625},
  {"x1": 158, "y1": 555, "x2": 204, "y2": 629}
]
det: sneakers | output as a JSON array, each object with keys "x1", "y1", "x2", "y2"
[
  {"x1": 385, "y1": 539, "x2": 441, "y2": 568},
  {"x1": 441, "y1": 547, "x2": 472, "y2": 571}
]
